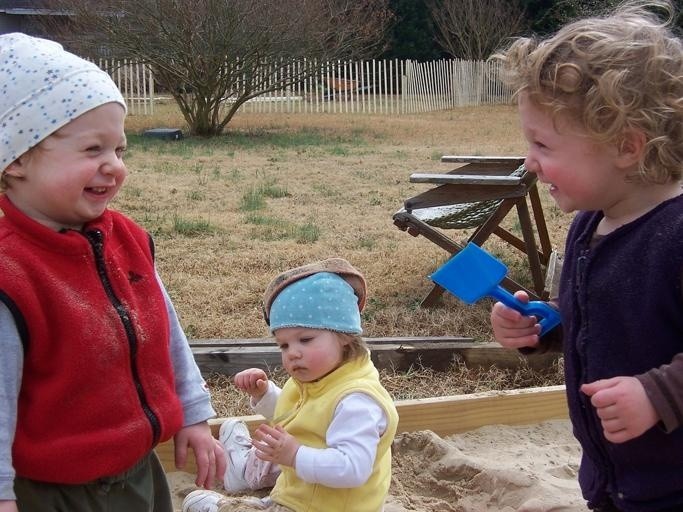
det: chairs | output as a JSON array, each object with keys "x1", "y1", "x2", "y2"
[{"x1": 393, "y1": 156, "x2": 555, "y2": 311}]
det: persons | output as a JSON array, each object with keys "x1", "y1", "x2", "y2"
[
  {"x1": 491, "y1": 0, "x2": 682, "y2": 512},
  {"x1": 181, "y1": 256, "x2": 400, "y2": 511},
  {"x1": 0, "y1": 32, "x2": 229, "y2": 511}
]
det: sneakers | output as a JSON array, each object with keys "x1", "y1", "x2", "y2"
[
  {"x1": 182, "y1": 487, "x2": 263, "y2": 512},
  {"x1": 216, "y1": 418, "x2": 252, "y2": 493}
]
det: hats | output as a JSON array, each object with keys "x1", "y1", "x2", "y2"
[
  {"x1": 265, "y1": 274, "x2": 365, "y2": 338},
  {"x1": 0, "y1": 28, "x2": 126, "y2": 172}
]
